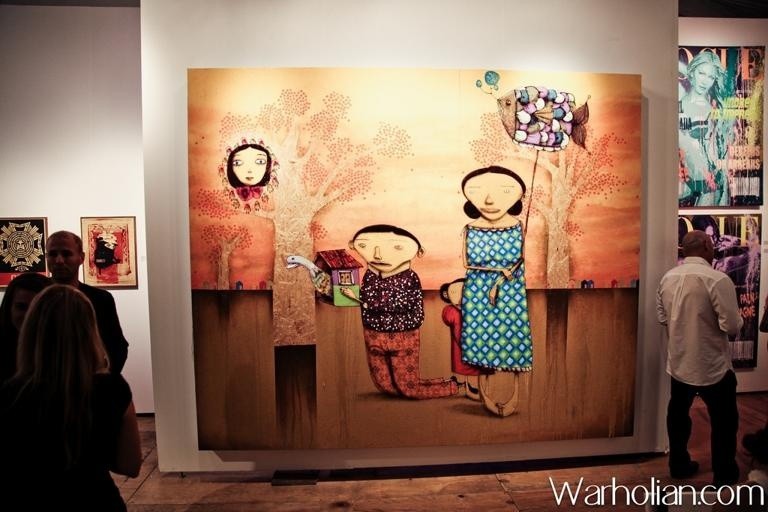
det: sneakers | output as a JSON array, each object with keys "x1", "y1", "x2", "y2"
[{"x1": 670, "y1": 459, "x2": 701, "y2": 481}]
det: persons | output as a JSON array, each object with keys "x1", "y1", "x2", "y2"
[
  {"x1": 691, "y1": 215, "x2": 749, "y2": 272},
  {"x1": 655, "y1": 229, "x2": 745, "y2": 488},
  {"x1": 0, "y1": 282, "x2": 142, "y2": 511},
  {"x1": 45, "y1": 228, "x2": 130, "y2": 375},
  {"x1": 1, "y1": 271, "x2": 57, "y2": 392},
  {"x1": 714, "y1": 423, "x2": 767, "y2": 511},
  {"x1": 680, "y1": 50, "x2": 737, "y2": 207}
]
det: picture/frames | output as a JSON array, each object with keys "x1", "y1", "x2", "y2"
[
  {"x1": 0, "y1": 217, "x2": 51, "y2": 288},
  {"x1": 80, "y1": 216, "x2": 138, "y2": 289}
]
